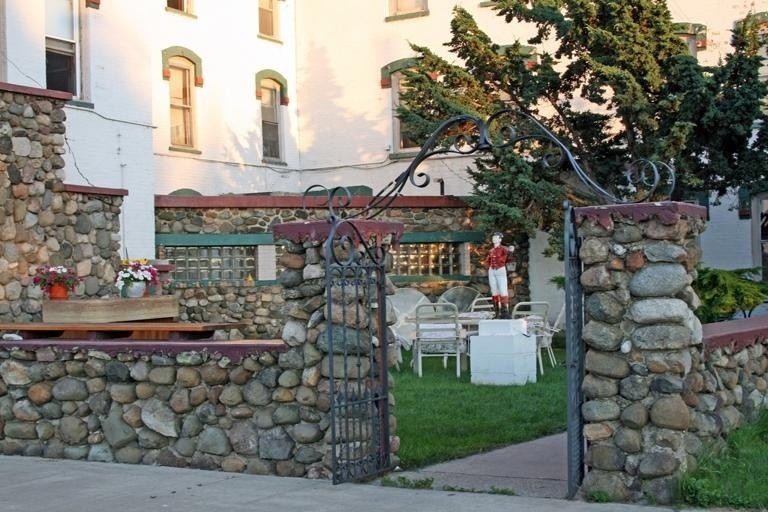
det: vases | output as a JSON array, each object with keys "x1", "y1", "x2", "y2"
[
  {"x1": 49, "y1": 284, "x2": 70, "y2": 301},
  {"x1": 126, "y1": 281, "x2": 147, "y2": 298}
]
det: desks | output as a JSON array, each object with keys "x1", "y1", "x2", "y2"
[
  {"x1": 1, "y1": 320, "x2": 239, "y2": 340},
  {"x1": 405, "y1": 311, "x2": 497, "y2": 372}
]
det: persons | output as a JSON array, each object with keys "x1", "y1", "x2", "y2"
[{"x1": 478, "y1": 232, "x2": 515, "y2": 319}]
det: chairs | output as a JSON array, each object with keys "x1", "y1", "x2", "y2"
[{"x1": 412, "y1": 296, "x2": 566, "y2": 379}]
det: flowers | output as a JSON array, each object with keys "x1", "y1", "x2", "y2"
[
  {"x1": 113, "y1": 256, "x2": 162, "y2": 300},
  {"x1": 31, "y1": 265, "x2": 82, "y2": 296}
]
